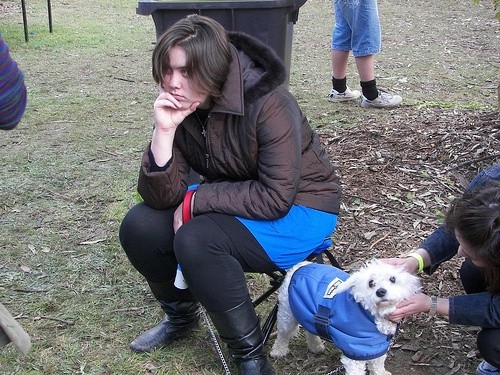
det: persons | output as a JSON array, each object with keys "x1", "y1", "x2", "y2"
[
  {"x1": 329, "y1": 0, "x2": 403, "y2": 107},
  {"x1": 0, "y1": 32, "x2": 27, "y2": 130},
  {"x1": 378, "y1": 163, "x2": 500, "y2": 375},
  {"x1": 119, "y1": 15, "x2": 341, "y2": 375}
]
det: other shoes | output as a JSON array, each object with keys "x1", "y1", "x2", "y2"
[{"x1": 477, "y1": 360, "x2": 500, "y2": 375}]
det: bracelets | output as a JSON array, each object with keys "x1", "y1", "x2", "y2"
[
  {"x1": 404, "y1": 252, "x2": 423, "y2": 274},
  {"x1": 424, "y1": 295, "x2": 437, "y2": 318}
]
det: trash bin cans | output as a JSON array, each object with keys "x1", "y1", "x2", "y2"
[{"x1": 135, "y1": 1, "x2": 305, "y2": 91}]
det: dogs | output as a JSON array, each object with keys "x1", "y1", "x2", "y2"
[{"x1": 269, "y1": 259, "x2": 421, "y2": 375}]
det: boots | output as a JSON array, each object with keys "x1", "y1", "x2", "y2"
[
  {"x1": 130, "y1": 277, "x2": 199, "y2": 352},
  {"x1": 211, "y1": 295, "x2": 277, "y2": 375}
]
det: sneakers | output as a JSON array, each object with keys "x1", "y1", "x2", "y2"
[
  {"x1": 361, "y1": 89, "x2": 403, "y2": 108},
  {"x1": 329, "y1": 84, "x2": 361, "y2": 102}
]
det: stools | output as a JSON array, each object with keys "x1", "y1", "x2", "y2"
[{"x1": 253, "y1": 237, "x2": 346, "y2": 345}]
